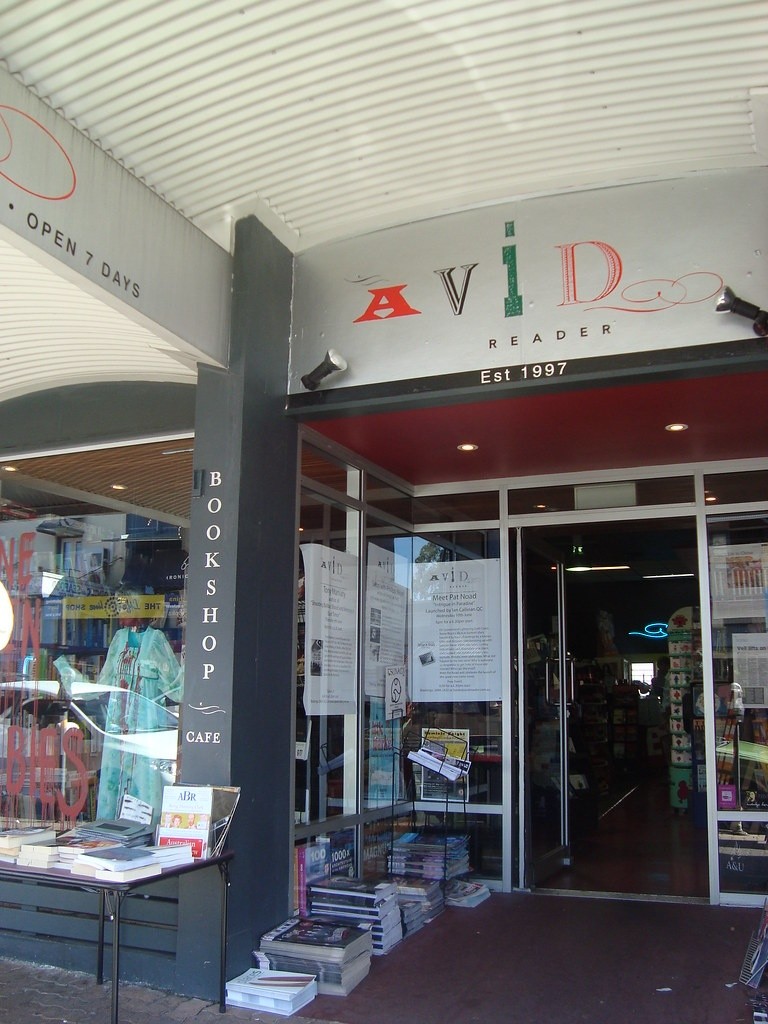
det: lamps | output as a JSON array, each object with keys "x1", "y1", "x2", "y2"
[
  {"x1": 716, "y1": 283, "x2": 768, "y2": 336},
  {"x1": 299, "y1": 348, "x2": 347, "y2": 391}
]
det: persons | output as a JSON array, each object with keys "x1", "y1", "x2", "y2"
[
  {"x1": 648, "y1": 670, "x2": 668, "y2": 698},
  {"x1": 53, "y1": 590, "x2": 184, "y2": 827},
  {"x1": 692, "y1": 653, "x2": 703, "y2": 699}
]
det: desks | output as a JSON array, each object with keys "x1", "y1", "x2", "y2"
[{"x1": 0, "y1": 836, "x2": 234, "y2": 1024}]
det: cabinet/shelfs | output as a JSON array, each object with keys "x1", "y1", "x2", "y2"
[
  {"x1": 666, "y1": 605, "x2": 728, "y2": 819},
  {"x1": 0, "y1": 593, "x2": 109, "y2": 821},
  {"x1": 578, "y1": 685, "x2": 641, "y2": 785}
]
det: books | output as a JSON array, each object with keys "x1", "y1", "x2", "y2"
[
  {"x1": 0, "y1": 785, "x2": 214, "y2": 883},
  {"x1": 407, "y1": 747, "x2": 471, "y2": 782},
  {"x1": 226, "y1": 832, "x2": 491, "y2": 1017}
]
